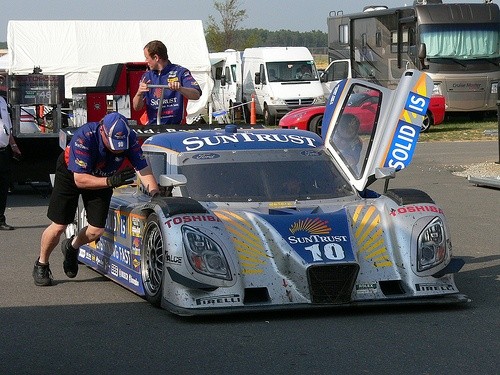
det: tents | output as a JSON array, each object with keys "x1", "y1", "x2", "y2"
[
  {"x1": 0, "y1": 54, "x2": 9, "y2": 71},
  {"x1": 7, "y1": 19, "x2": 214, "y2": 123}
]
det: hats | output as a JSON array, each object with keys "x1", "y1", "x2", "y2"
[{"x1": 103, "y1": 112, "x2": 132, "y2": 150}]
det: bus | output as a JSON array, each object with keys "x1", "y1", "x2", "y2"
[{"x1": 327, "y1": 0, "x2": 500, "y2": 118}]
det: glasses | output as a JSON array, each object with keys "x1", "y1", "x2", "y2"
[{"x1": 337, "y1": 131, "x2": 355, "y2": 143}]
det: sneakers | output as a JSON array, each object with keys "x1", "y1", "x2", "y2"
[
  {"x1": 61, "y1": 235, "x2": 78, "y2": 278},
  {"x1": 33, "y1": 256, "x2": 54, "y2": 286}
]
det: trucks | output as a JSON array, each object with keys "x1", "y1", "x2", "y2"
[{"x1": 210, "y1": 46, "x2": 352, "y2": 125}]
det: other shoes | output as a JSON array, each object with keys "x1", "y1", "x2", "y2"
[{"x1": 0, "y1": 221, "x2": 14, "y2": 231}]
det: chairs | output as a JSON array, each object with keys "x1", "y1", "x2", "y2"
[{"x1": 269, "y1": 69, "x2": 279, "y2": 81}]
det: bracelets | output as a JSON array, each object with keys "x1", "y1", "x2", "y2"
[{"x1": 10, "y1": 143, "x2": 18, "y2": 148}]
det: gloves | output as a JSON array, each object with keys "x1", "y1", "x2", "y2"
[{"x1": 106, "y1": 166, "x2": 136, "y2": 189}]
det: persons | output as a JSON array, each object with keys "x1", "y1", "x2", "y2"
[
  {"x1": 295, "y1": 65, "x2": 311, "y2": 79},
  {"x1": 334, "y1": 112, "x2": 363, "y2": 177},
  {"x1": 0, "y1": 97, "x2": 22, "y2": 230},
  {"x1": 132, "y1": 40, "x2": 202, "y2": 125},
  {"x1": 31, "y1": 113, "x2": 161, "y2": 286}
]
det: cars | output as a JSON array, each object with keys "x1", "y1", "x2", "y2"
[{"x1": 53, "y1": 69, "x2": 472, "y2": 317}]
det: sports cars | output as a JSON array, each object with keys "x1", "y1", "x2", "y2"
[{"x1": 277, "y1": 87, "x2": 445, "y2": 140}]
